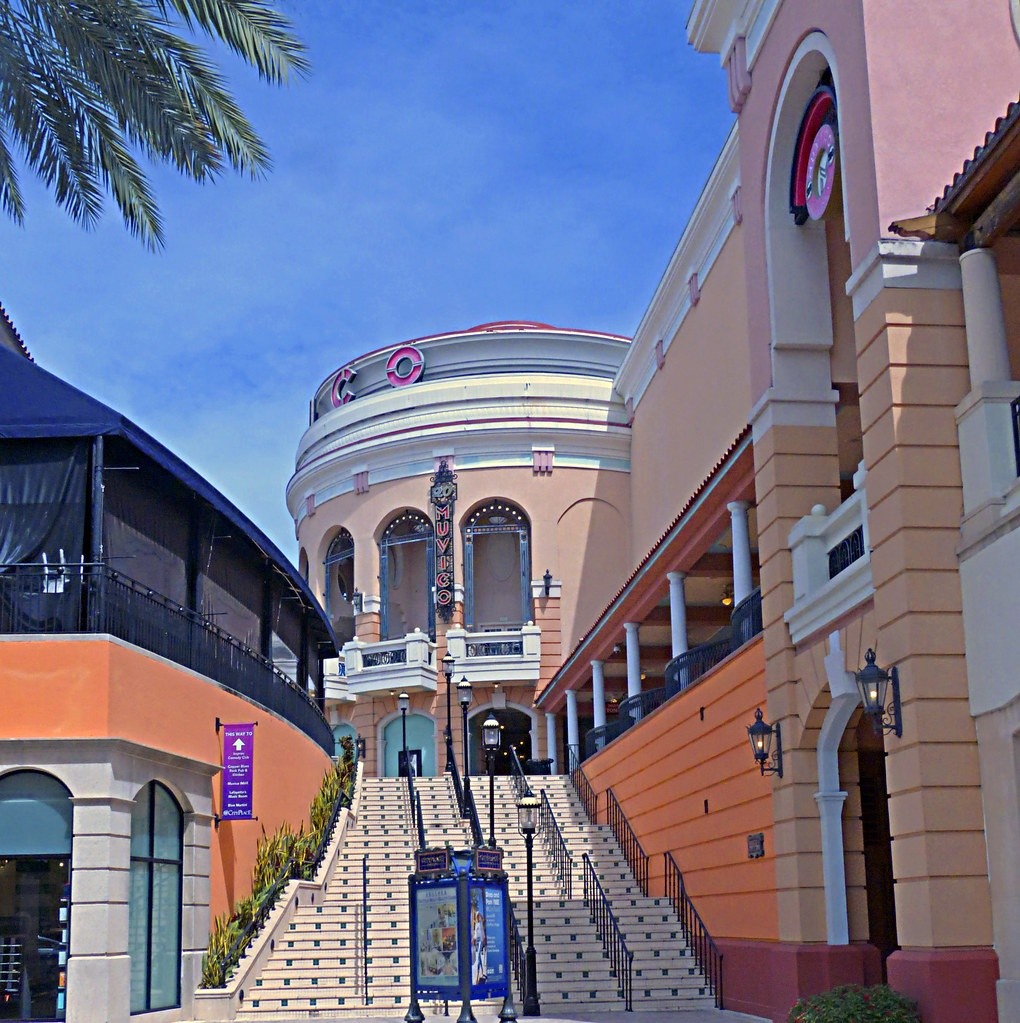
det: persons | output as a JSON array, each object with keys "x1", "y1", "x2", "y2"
[{"x1": 472, "y1": 911, "x2": 486, "y2": 982}]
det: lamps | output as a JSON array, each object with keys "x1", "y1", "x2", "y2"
[
  {"x1": 353, "y1": 587, "x2": 363, "y2": 612},
  {"x1": 720, "y1": 585, "x2": 732, "y2": 605},
  {"x1": 852, "y1": 648, "x2": 901, "y2": 739},
  {"x1": 640, "y1": 669, "x2": 646, "y2": 680},
  {"x1": 610, "y1": 694, "x2": 618, "y2": 703},
  {"x1": 746, "y1": 706, "x2": 783, "y2": 779},
  {"x1": 544, "y1": 569, "x2": 552, "y2": 596}
]
